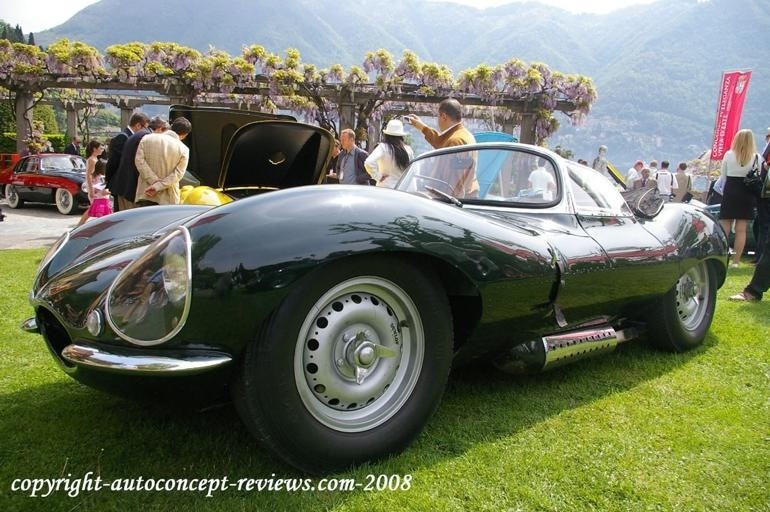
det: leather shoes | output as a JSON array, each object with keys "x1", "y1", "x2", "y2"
[{"x1": 730, "y1": 292, "x2": 756, "y2": 300}]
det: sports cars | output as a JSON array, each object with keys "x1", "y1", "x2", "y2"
[
  {"x1": 0, "y1": 153, "x2": 90, "y2": 214},
  {"x1": 681, "y1": 180, "x2": 759, "y2": 254},
  {"x1": 21, "y1": 142, "x2": 730, "y2": 473}
]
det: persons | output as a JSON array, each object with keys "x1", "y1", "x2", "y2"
[
  {"x1": 326, "y1": 138, "x2": 341, "y2": 184},
  {"x1": 62, "y1": 112, "x2": 192, "y2": 228},
  {"x1": 526, "y1": 129, "x2": 770, "y2": 300},
  {"x1": 363, "y1": 119, "x2": 416, "y2": 192},
  {"x1": 335, "y1": 128, "x2": 373, "y2": 185},
  {"x1": 408, "y1": 98, "x2": 480, "y2": 200}
]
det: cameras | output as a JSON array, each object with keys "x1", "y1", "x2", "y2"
[
  {"x1": 744, "y1": 177, "x2": 759, "y2": 187},
  {"x1": 402, "y1": 116, "x2": 414, "y2": 125}
]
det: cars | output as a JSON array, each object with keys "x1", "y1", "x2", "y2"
[
  {"x1": 166, "y1": 104, "x2": 336, "y2": 209},
  {"x1": 471, "y1": 131, "x2": 519, "y2": 201}
]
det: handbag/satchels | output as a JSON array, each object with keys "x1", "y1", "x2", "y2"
[{"x1": 744, "y1": 154, "x2": 763, "y2": 193}]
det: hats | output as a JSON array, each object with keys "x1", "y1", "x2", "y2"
[{"x1": 382, "y1": 120, "x2": 405, "y2": 136}]
